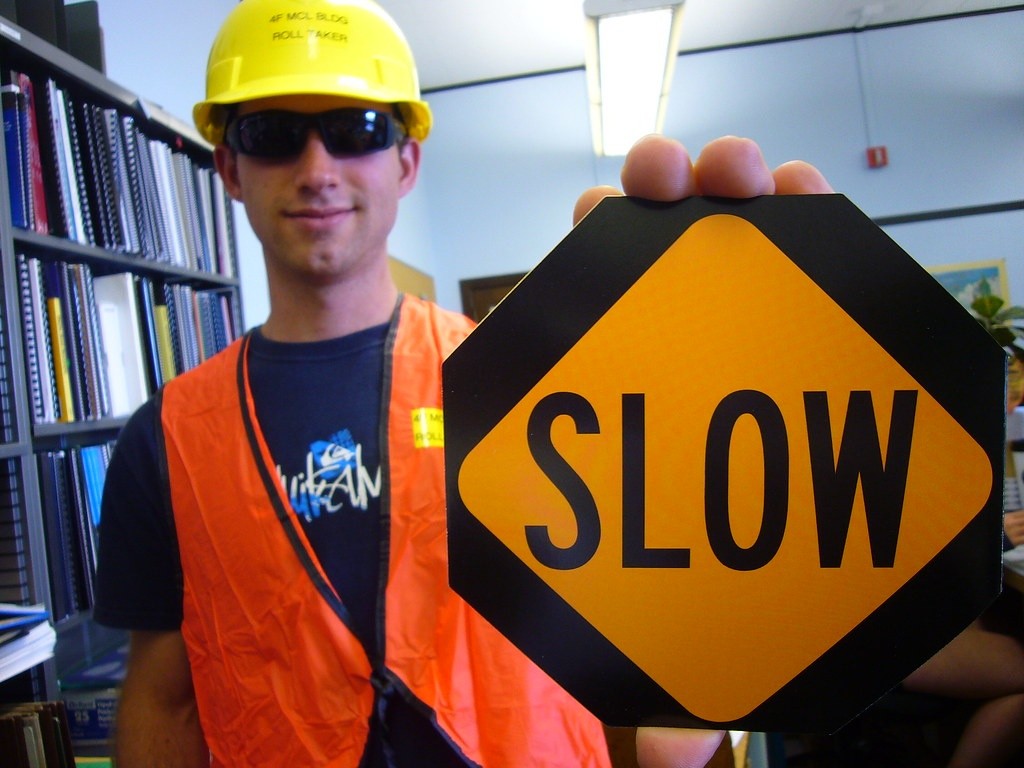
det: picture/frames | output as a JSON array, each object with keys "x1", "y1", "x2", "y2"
[{"x1": 927, "y1": 257, "x2": 1013, "y2": 326}]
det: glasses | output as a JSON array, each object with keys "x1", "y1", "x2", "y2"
[{"x1": 225, "y1": 107, "x2": 407, "y2": 162}]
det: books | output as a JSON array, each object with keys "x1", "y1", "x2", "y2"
[{"x1": 1, "y1": 64, "x2": 235, "y2": 768}]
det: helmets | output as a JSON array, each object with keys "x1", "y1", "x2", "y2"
[{"x1": 193, "y1": 0, "x2": 432, "y2": 146}]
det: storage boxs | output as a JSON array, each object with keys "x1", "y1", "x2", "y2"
[{"x1": 59, "y1": 641, "x2": 128, "y2": 756}]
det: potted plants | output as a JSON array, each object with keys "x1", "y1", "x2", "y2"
[{"x1": 970, "y1": 295, "x2": 1024, "y2": 416}]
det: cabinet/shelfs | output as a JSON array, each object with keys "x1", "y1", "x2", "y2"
[{"x1": 0, "y1": 12, "x2": 246, "y2": 698}]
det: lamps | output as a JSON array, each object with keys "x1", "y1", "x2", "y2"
[{"x1": 583, "y1": 0, "x2": 688, "y2": 159}]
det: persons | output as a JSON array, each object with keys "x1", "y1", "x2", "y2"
[{"x1": 96, "y1": 1, "x2": 1024, "y2": 768}]
